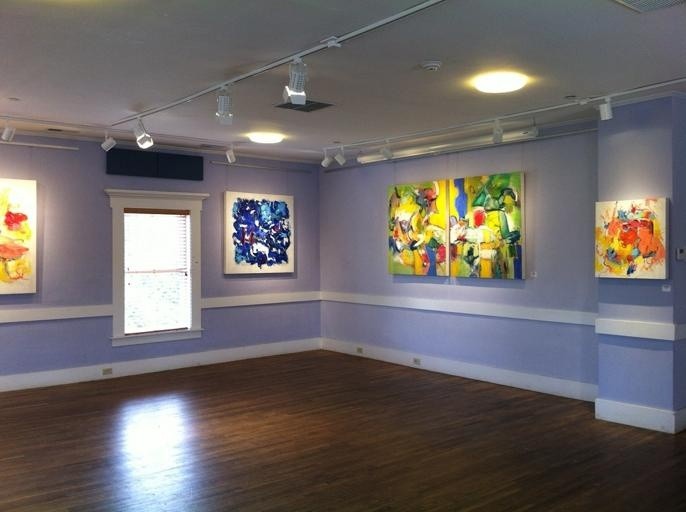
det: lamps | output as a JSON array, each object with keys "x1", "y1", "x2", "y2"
[
  {"x1": 100, "y1": 131, "x2": 118, "y2": 152},
  {"x1": 1, "y1": 123, "x2": 16, "y2": 143},
  {"x1": 598, "y1": 96, "x2": 614, "y2": 121},
  {"x1": 132, "y1": 117, "x2": 154, "y2": 150},
  {"x1": 492, "y1": 118, "x2": 504, "y2": 144},
  {"x1": 519, "y1": 116, "x2": 539, "y2": 139},
  {"x1": 320, "y1": 145, "x2": 347, "y2": 169},
  {"x1": 225, "y1": 148, "x2": 236, "y2": 164},
  {"x1": 216, "y1": 86, "x2": 234, "y2": 121},
  {"x1": 380, "y1": 144, "x2": 394, "y2": 160},
  {"x1": 281, "y1": 56, "x2": 309, "y2": 107}
]
